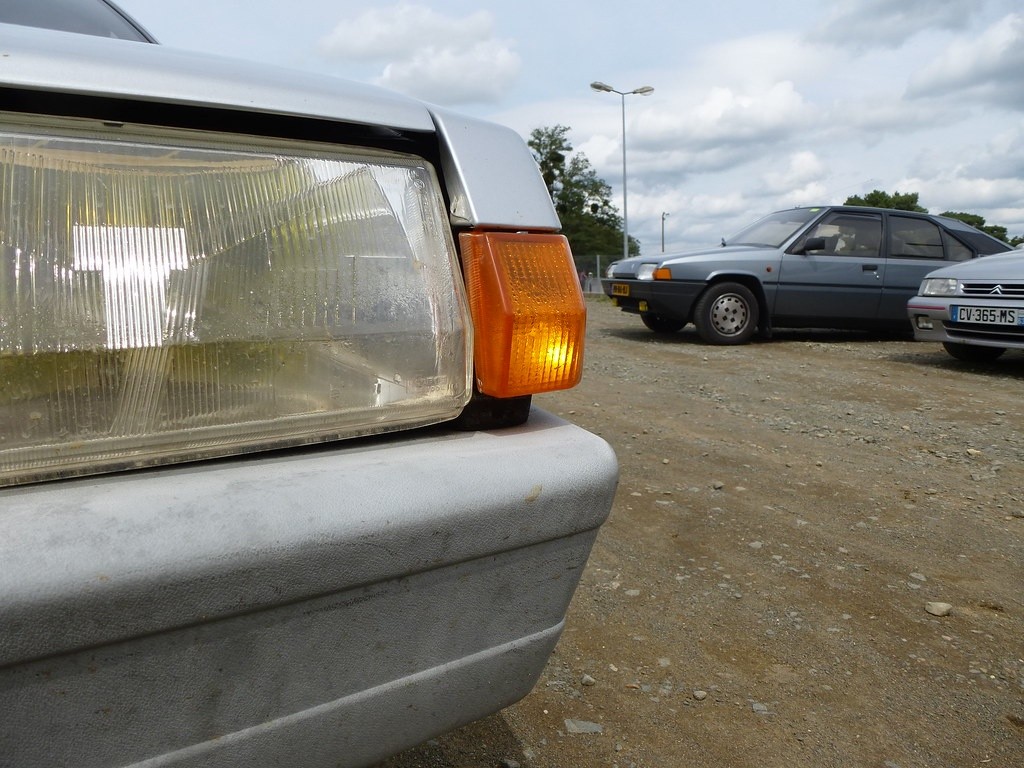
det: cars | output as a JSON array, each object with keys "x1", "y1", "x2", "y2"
[
  {"x1": 602, "y1": 206, "x2": 1013, "y2": 343},
  {"x1": 0, "y1": 1, "x2": 616, "y2": 765},
  {"x1": 908, "y1": 242, "x2": 1024, "y2": 360}
]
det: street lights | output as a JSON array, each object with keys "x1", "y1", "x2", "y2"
[
  {"x1": 662, "y1": 212, "x2": 671, "y2": 252},
  {"x1": 590, "y1": 82, "x2": 654, "y2": 258}
]
